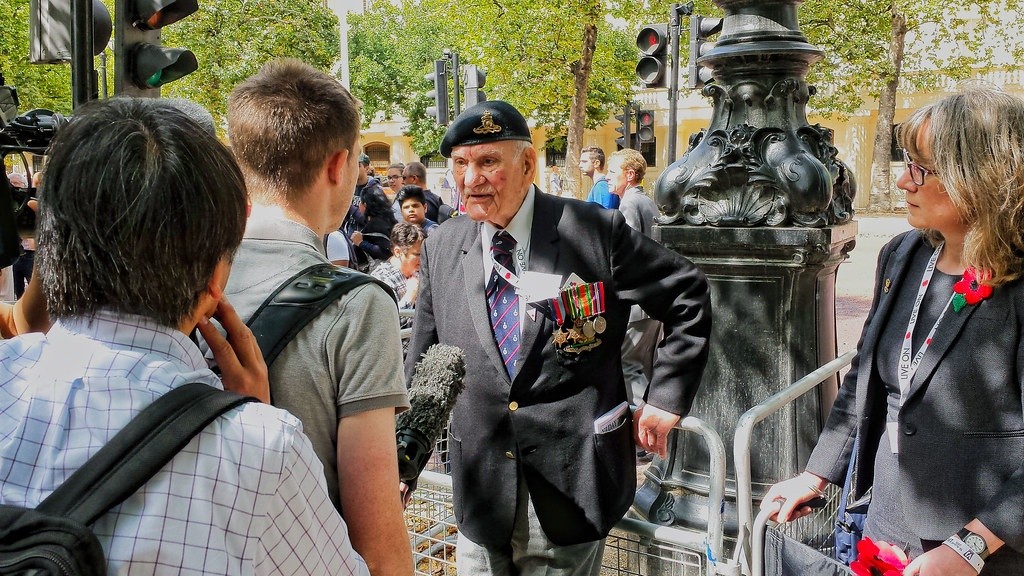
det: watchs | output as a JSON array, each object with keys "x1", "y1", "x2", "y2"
[{"x1": 957, "y1": 528, "x2": 990, "y2": 560}]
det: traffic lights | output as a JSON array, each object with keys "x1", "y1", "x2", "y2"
[
  {"x1": 426, "y1": 61, "x2": 449, "y2": 127},
  {"x1": 114, "y1": 0, "x2": 198, "y2": 100},
  {"x1": 688, "y1": 15, "x2": 724, "y2": 90},
  {"x1": 615, "y1": 105, "x2": 630, "y2": 149},
  {"x1": 29, "y1": 0, "x2": 114, "y2": 62},
  {"x1": 636, "y1": 22, "x2": 669, "y2": 90},
  {"x1": 464, "y1": 64, "x2": 487, "y2": 110},
  {"x1": 637, "y1": 110, "x2": 655, "y2": 144}
]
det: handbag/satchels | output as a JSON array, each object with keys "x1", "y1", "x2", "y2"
[{"x1": 361, "y1": 232, "x2": 390, "y2": 274}]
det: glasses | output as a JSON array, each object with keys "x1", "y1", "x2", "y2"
[
  {"x1": 902, "y1": 147, "x2": 939, "y2": 186},
  {"x1": 387, "y1": 175, "x2": 402, "y2": 181}
]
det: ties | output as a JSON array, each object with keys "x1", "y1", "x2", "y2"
[{"x1": 485, "y1": 231, "x2": 521, "y2": 380}]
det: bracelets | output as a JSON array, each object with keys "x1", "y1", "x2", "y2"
[
  {"x1": 794, "y1": 473, "x2": 824, "y2": 498},
  {"x1": 942, "y1": 537, "x2": 985, "y2": 575}
]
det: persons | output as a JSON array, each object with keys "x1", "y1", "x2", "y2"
[
  {"x1": 550, "y1": 166, "x2": 561, "y2": 196},
  {"x1": 760, "y1": 86, "x2": 1024, "y2": 576},
  {"x1": 0, "y1": 154, "x2": 463, "y2": 347},
  {"x1": 405, "y1": 101, "x2": 712, "y2": 576},
  {"x1": 0, "y1": 94, "x2": 371, "y2": 576},
  {"x1": 579, "y1": 147, "x2": 620, "y2": 210},
  {"x1": 606, "y1": 148, "x2": 661, "y2": 407},
  {"x1": 191, "y1": 63, "x2": 413, "y2": 576}
]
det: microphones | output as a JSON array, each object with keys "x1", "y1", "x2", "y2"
[
  {"x1": 119, "y1": 96, "x2": 216, "y2": 136},
  {"x1": 395, "y1": 344, "x2": 467, "y2": 491}
]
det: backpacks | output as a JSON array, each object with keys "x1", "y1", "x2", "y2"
[
  {"x1": 0, "y1": 381, "x2": 261, "y2": 576},
  {"x1": 426, "y1": 192, "x2": 458, "y2": 225}
]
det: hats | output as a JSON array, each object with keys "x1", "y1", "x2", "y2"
[
  {"x1": 439, "y1": 101, "x2": 533, "y2": 159},
  {"x1": 358, "y1": 153, "x2": 370, "y2": 166}
]
